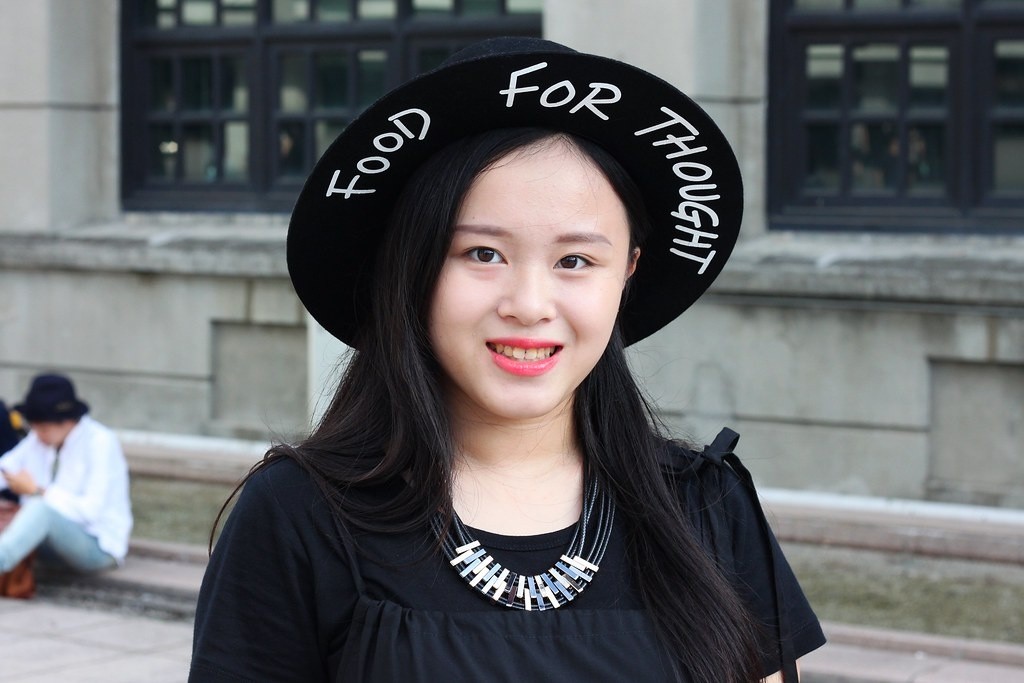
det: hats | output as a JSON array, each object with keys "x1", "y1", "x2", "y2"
[
  {"x1": 13, "y1": 375, "x2": 89, "y2": 421},
  {"x1": 286, "y1": 35, "x2": 745, "y2": 354}
]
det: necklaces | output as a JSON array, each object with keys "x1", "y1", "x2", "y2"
[{"x1": 417, "y1": 452, "x2": 618, "y2": 612}]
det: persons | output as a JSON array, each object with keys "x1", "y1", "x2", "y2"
[
  {"x1": 188, "y1": 36, "x2": 827, "y2": 683},
  {"x1": 0, "y1": 374, "x2": 133, "y2": 579}
]
